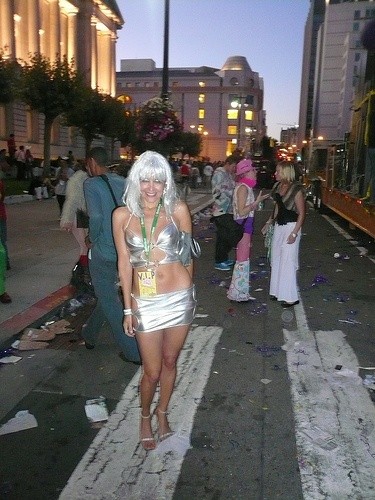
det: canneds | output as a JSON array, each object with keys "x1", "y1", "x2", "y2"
[{"x1": 258, "y1": 202, "x2": 264, "y2": 209}]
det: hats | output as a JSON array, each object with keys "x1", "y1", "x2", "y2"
[{"x1": 235, "y1": 158, "x2": 255, "y2": 175}]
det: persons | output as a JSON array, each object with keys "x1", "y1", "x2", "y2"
[
  {"x1": 261, "y1": 160, "x2": 307, "y2": 307},
  {"x1": 49, "y1": 155, "x2": 75, "y2": 219},
  {"x1": 211, "y1": 155, "x2": 245, "y2": 271},
  {"x1": 227, "y1": 159, "x2": 271, "y2": 302},
  {"x1": 170, "y1": 157, "x2": 225, "y2": 188},
  {"x1": 16, "y1": 146, "x2": 34, "y2": 180},
  {"x1": 61, "y1": 166, "x2": 89, "y2": 290},
  {"x1": 112, "y1": 150, "x2": 199, "y2": 451},
  {"x1": 7, "y1": 133, "x2": 17, "y2": 164},
  {"x1": 0, "y1": 147, "x2": 13, "y2": 179},
  {"x1": 56, "y1": 150, "x2": 78, "y2": 171},
  {"x1": 31, "y1": 176, "x2": 54, "y2": 201},
  {"x1": 76, "y1": 147, "x2": 142, "y2": 366}
]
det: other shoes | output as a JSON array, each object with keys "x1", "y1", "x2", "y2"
[
  {"x1": 119, "y1": 352, "x2": 143, "y2": 365},
  {"x1": 214, "y1": 262, "x2": 230, "y2": 271},
  {"x1": 223, "y1": 259, "x2": 234, "y2": 266},
  {"x1": 270, "y1": 296, "x2": 277, "y2": 301},
  {"x1": 281, "y1": 300, "x2": 299, "y2": 308},
  {"x1": 0, "y1": 292, "x2": 13, "y2": 304}
]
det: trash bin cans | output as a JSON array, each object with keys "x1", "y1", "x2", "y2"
[{"x1": 174, "y1": 174, "x2": 188, "y2": 201}]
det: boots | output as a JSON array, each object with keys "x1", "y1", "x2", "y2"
[{"x1": 71, "y1": 263, "x2": 96, "y2": 297}]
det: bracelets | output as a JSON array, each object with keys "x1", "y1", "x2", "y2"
[
  {"x1": 248, "y1": 202, "x2": 255, "y2": 211},
  {"x1": 123, "y1": 309, "x2": 132, "y2": 316},
  {"x1": 291, "y1": 232, "x2": 298, "y2": 238}
]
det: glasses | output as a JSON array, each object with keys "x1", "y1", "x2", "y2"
[{"x1": 140, "y1": 178, "x2": 164, "y2": 187}]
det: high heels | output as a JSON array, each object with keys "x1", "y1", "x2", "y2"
[
  {"x1": 155, "y1": 407, "x2": 173, "y2": 442},
  {"x1": 140, "y1": 412, "x2": 156, "y2": 450}
]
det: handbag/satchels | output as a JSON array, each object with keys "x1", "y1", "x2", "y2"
[{"x1": 210, "y1": 213, "x2": 244, "y2": 249}]
